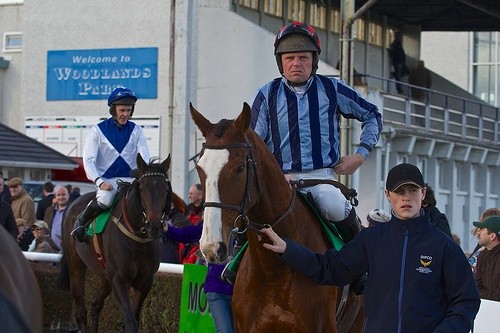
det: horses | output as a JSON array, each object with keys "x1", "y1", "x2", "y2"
[
  {"x1": 51, "y1": 151, "x2": 173, "y2": 333},
  {"x1": 187, "y1": 99, "x2": 370, "y2": 333}
]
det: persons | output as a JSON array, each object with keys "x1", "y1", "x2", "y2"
[
  {"x1": 256, "y1": 163, "x2": 482, "y2": 333},
  {"x1": 178, "y1": 183, "x2": 204, "y2": 265},
  {"x1": 0, "y1": 172, "x2": 83, "y2": 254},
  {"x1": 409, "y1": 60, "x2": 432, "y2": 128},
  {"x1": 335, "y1": 59, "x2": 363, "y2": 86},
  {"x1": 248, "y1": 21, "x2": 383, "y2": 296},
  {"x1": 421, "y1": 182, "x2": 454, "y2": 240},
  {"x1": 163, "y1": 208, "x2": 239, "y2": 333},
  {"x1": 389, "y1": 31, "x2": 410, "y2": 97},
  {"x1": 71, "y1": 87, "x2": 154, "y2": 243},
  {"x1": 468, "y1": 208, "x2": 500, "y2": 302}
]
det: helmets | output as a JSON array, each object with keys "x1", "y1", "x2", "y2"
[
  {"x1": 107, "y1": 87, "x2": 138, "y2": 106},
  {"x1": 274, "y1": 22, "x2": 321, "y2": 54}
]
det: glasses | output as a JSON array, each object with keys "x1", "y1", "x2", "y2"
[{"x1": 31, "y1": 228, "x2": 40, "y2": 231}]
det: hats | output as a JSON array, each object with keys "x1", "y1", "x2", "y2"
[
  {"x1": 472, "y1": 215, "x2": 500, "y2": 235},
  {"x1": 7, "y1": 177, "x2": 23, "y2": 187},
  {"x1": 369, "y1": 208, "x2": 391, "y2": 223},
  {"x1": 386, "y1": 163, "x2": 424, "y2": 192},
  {"x1": 33, "y1": 220, "x2": 48, "y2": 229}
]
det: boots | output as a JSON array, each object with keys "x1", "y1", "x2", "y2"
[
  {"x1": 330, "y1": 207, "x2": 368, "y2": 295},
  {"x1": 73, "y1": 198, "x2": 104, "y2": 243}
]
